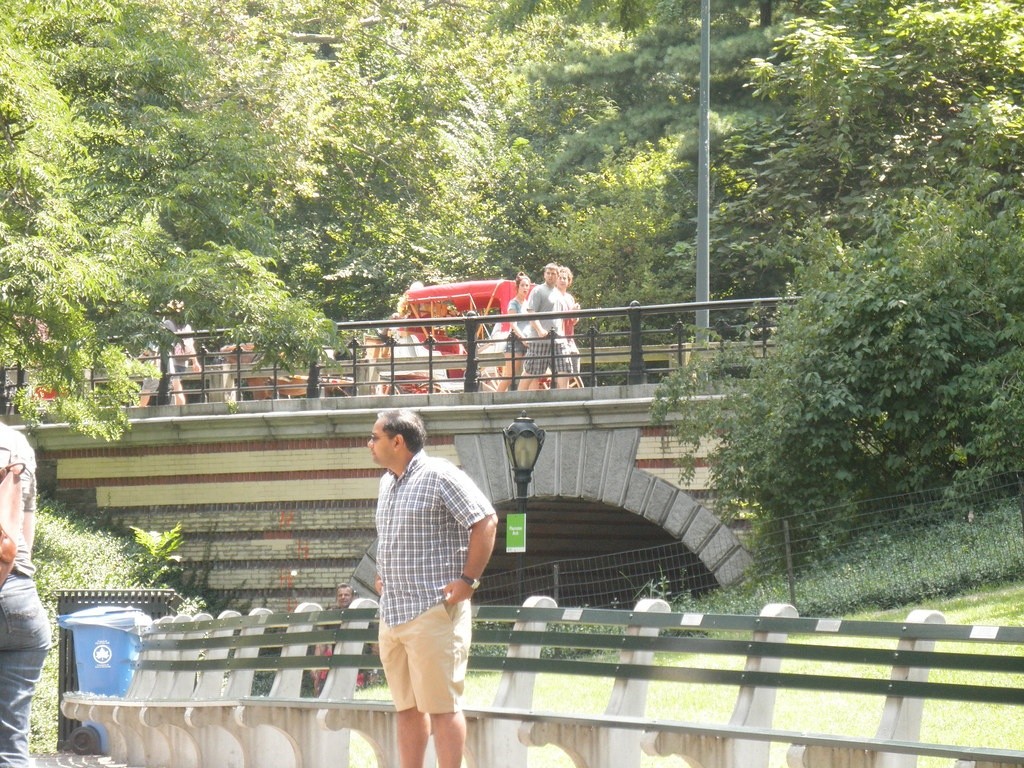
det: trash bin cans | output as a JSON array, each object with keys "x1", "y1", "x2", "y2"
[{"x1": 56, "y1": 604, "x2": 152, "y2": 758}]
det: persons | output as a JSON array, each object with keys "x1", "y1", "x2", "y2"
[
  {"x1": 0, "y1": 420, "x2": 52, "y2": 768},
  {"x1": 312, "y1": 584, "x2": 380, "y2": 697},
  {"x1": 497, "y1": 271, "x2": 531, "y2": 392},
  {"x1": 139, "y1": 301, "x2": 200, "y2": 406},
  {"x1": 529, "y1": 266, "x2": 581, "y2": 390},
  {"x1": 518, "y1": 262, "x2": 572, "y2": 391},
  {"x1": 368, "y1": 410, "x2": 498, "y2": 768}
]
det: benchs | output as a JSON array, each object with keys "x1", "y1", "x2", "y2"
[{"x1": 411, "y1": 333, "x2": 467, "y2": 378}]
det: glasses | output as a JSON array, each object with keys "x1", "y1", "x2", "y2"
[{"x1": 369, "y1": 431, "x2": 393, "y2": 441}]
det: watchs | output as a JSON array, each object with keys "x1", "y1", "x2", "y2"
[{"x1": 461, "y1": 573, "x2": 480, "y2": 589}]
